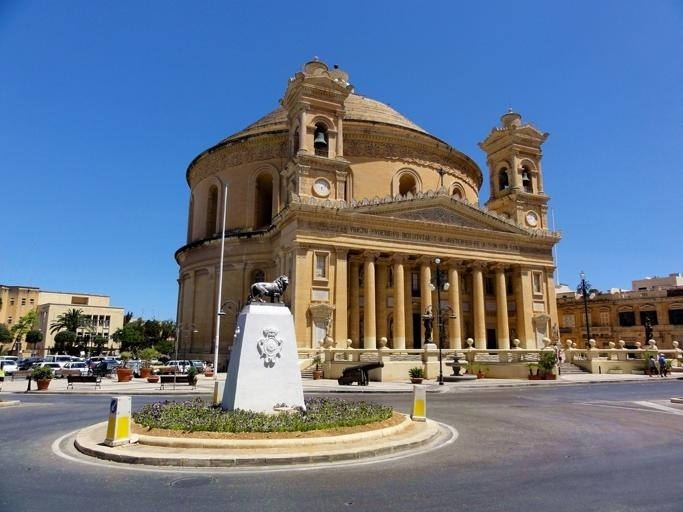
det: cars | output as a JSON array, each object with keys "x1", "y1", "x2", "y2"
[{"x1": 1, "y1": 353, "x2": 216, "y2": 385}]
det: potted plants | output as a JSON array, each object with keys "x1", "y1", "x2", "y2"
[
  {"x1": 313, "y1": 352, "x2": 324, "y2": 379},
  {"x1": 643, "y1": 351, "x2": 655, "y2": 374},
  {"x1": 666, "y1": 360, "x2": 673, "y2": 375},
  {"x1": 524, "y1": 351, "x2": 558, "y2": 380},
  {"x1": 408, "y1": 366, "x2": 424, "y2": 383},
  {"x1": 136, "y1": 346, "x2": 162, "y2": 377},
  {"x1": 32, "y1": 365, "x2": 54, "y2": 390},
  {"x1": 116, "y1": 351, "x2": 133, "y2": 382}
]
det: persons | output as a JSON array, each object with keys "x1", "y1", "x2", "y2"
[
  {"x1": 643, "y1": 317, "x2": 653, "y2": 345},
  {"x1": 201, "y1": 360, "x2": 206, "y2": 374},
  {"x1": 0, "y1": 362, "x2": 5, "y2": 393},
  {"x1": 422, "y1": 310, "x2": 435, "y2": 344},
  {"x1": 648, "y1": 355, "x2": 658, "y2": 376},
  {"x1": 657, "y1": 352, "x2": 667, "y2": 377}
]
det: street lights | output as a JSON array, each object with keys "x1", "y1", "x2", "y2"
[
  {"x1": 216, "y1": 297, "x2": 242, "y2": 326},
  {"x1": 428, "y1": 252, "x2": 448, "y2": 348},
  {"x1": 644, "y1": 315, "x2": 654, "y2": 348},
  {"x1": 170, "y1": 321, "x2": 202, "y2": 389},
  {"x1": 420, "y1": 305, "x2": 459, "y2": 388},
  {"x1": 575, "y1": 270, "x2": 597, "y2": 353}
]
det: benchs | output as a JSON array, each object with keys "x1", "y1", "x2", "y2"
[
  {"x1": 67, "y1": 375, "x2": 98, "y2": 382},
  {"x1": 161, "y1": 375, "x2": 188, "y2": 382}
]
